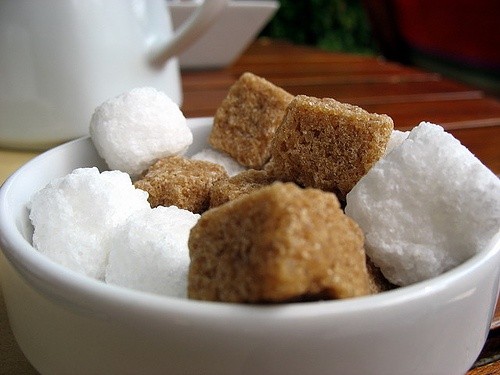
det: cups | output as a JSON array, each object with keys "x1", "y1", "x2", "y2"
[{"x1": 0, "y1": 0, "x2": 230, "y2": 152}]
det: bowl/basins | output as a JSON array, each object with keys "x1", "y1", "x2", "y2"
[
  {"x1": 168, "y1": 0, "x2": 279, "y2": 68},
  {"x1": 0, "y1": 116, "x2": 500, "y2": 375}
]
